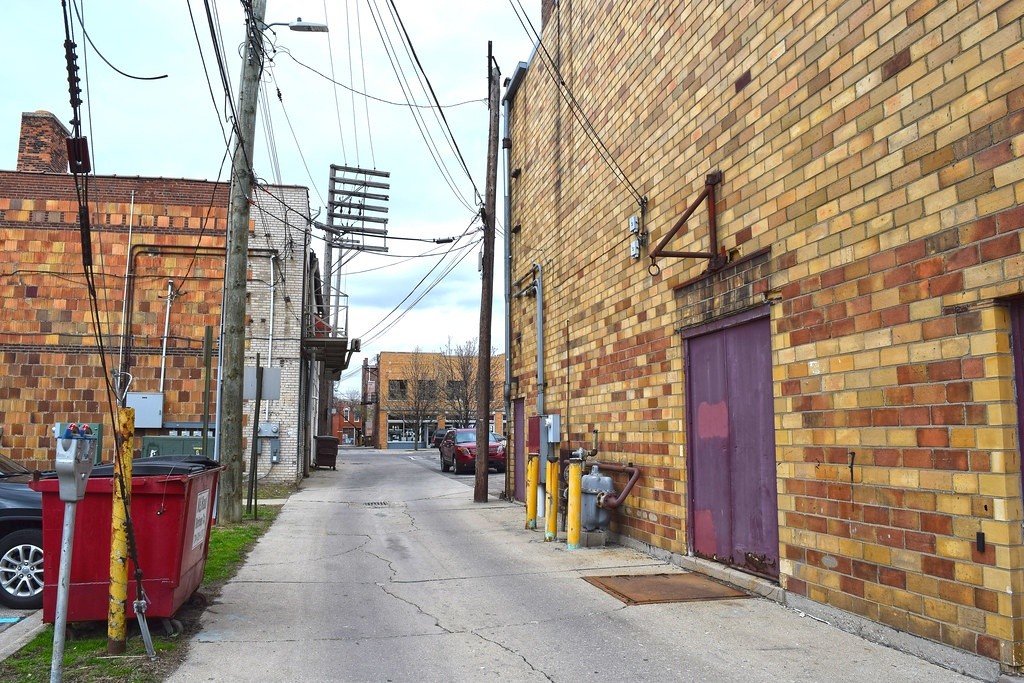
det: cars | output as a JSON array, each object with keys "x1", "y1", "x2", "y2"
[
  {"x1": 0, "y1": 452, "x2": 44, "y2": 610},
  {"x1": 492, "y1": 433, "x2": 506, "y2": 441},
  {"x1": 431, "y1": 429, "x2": 447, "y2": 448}
]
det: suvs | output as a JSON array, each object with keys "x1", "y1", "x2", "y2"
[{"x1": 439, "y1": 428, "x2": 505, "y2": 475}]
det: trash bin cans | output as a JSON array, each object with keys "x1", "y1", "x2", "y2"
[
  {"x1": 313, "y1": 436, "x2": 339, "y2": 470},
  {"x1": 28, "y1": 454, "x2": 227, "y2": 638}
]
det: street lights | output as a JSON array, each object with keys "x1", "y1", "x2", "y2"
[{"x1": 217, "y1": 21, "x2": 328, "y2": 525}]
